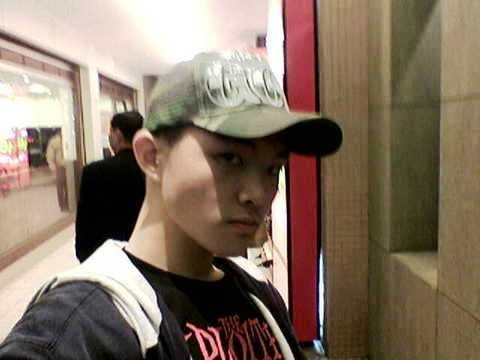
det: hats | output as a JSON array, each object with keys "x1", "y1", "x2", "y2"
[{"x1": 141, "y1": 51, "x2": 343, "y2": 157}]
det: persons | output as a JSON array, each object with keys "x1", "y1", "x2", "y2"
[
  {"x1": 1, "y1": 49, "x2": 343, "y2": 360},
  {"x1": 75, "y1": 108, "x2": 147, "y2": 269},
  {"x1": 45, "y1": 129, "x2": 69, "y2": 213}
]
